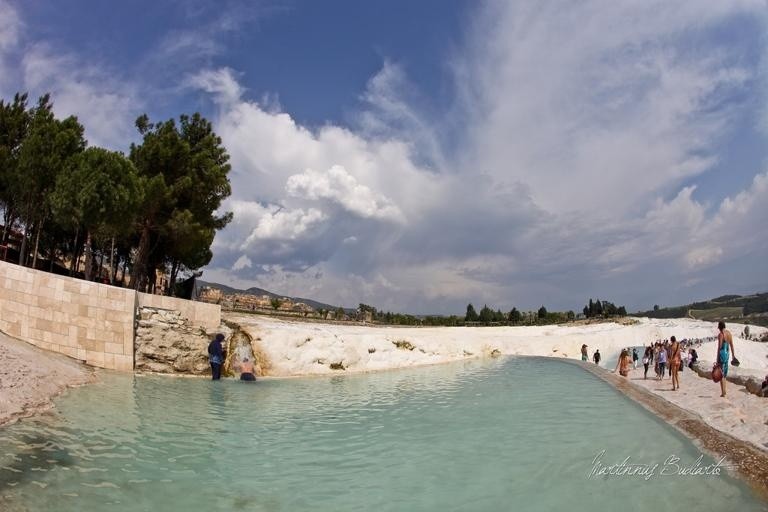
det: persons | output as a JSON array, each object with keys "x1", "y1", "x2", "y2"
[
  {"x1": 230, "y1": 359, "x2": 259, "y2": 382},
  {"x1": 581, "y1": 318, "x2": 750, "y2": 397},
  {"x1": 208, "y1": 334, "x2": 227, "y2": 380}
]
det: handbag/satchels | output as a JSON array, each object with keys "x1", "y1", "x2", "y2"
[{"x1": 711, "y1": 364, "x2": 723, "y2": 383}]
